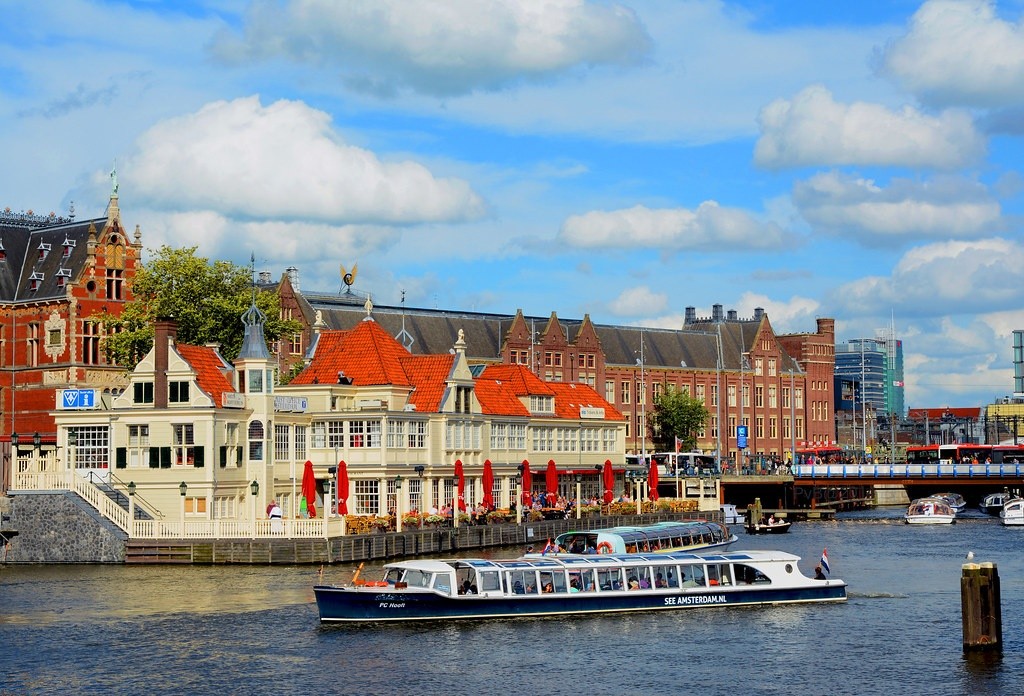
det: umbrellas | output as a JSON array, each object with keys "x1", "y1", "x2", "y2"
[
  {"x1": 522, "y1": 460, "x2": 558, "y2": 505},
  {"x1": 602, "y1": 459, "x2": 659, "y2": 503},
  {"x1": 301, "y1": 460, "x2": 349, "y2": 515},
  {"x1": 454, "y1": 459, "x2": 494, "y2": 512}
]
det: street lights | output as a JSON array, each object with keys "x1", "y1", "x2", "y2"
[
  {"x1": 33, "y1": 432, "x2": 42, "y2": 489},
  {"x1": 452, "y1": 474, "x2": 460, "y2": 526},
  {"x1": 394, "y1": 475, "x2": 403, "y2": 532},
  {"x1": 698, "y1": 470, "x2": 704, "y2": 499},
  {"x1": 681, "y1": 469, "x2": 686, "y2": 501},
  {"x1": 575, "y1": 472, "x2": 584, "y2": 519},
  {"x1": 642, "y1": 471, "x2": 649, "y2": 512},
  {"x1": 10, "y1": 431, "x2": 19, "y2": 491},
  {"x1": 249, "y1": 480, "x2": 259, "y2": 538},
  {"x1": 179, "y1": 481, "x2": 188, "y2": 538},
  {"x1": 516, "y1": 473, "x2": 522, "y2": 524},
  {"x1": 635, "y1": 471, "x2": 642, "y2": 514},
  {"x1": 127, "y1": 481, "x2": 136, "y2": 537},
  {"x1": 322, "y1": 479, "x2": 331, "y2": 538},
  {"x1": 715, "y1": 470, "x2": 721, "y2": 511}
]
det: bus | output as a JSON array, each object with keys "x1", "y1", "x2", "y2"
[
  {"x1": 905, "y1": 443, "x2": 1024, "y2": 464},
  {"x1": 625, "y1": 452, "x2": 717, "y2": 479},
  {"x1": 787, "y1": 446, "x2": 844, "y2": 467}
]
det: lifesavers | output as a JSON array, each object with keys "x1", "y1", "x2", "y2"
[
  {"x1": 597, "y1": 542, "x2": 613, "y2": 554},
  {"x1": 364, "y1": 581, "x2": 388, "y2": 588}
]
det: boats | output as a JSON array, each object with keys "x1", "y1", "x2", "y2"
[
  {"x1": 978, "y1": 489, "x2": 1021, "y2": 515},
  {"x1": 312, "y1": 552, "x2": 849, "y2": 625},
  {"x1": 928, "y1": 492, "x2": 969, "y2": 513},
  {"x1": 904, "y1": 497, "x2": 958, "y2": 524},
  {"x1": 553, "y1": 519, "x2": 739, "y2": 555},
  {"x1": 998, "y1": 494, "x2": 1024, "y2": 526},
  {"x1": 743, "y1": 522, "x2": 794, "y2": 536}
]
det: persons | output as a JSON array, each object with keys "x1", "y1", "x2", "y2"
[
  {"x1": 488, "y1": 505, "x2": 495, "y2": 511},
  {"x1": 387, "y1": 502, "x2": 486, "y2": 523},
  {"x1": 626, "y1": 533, "x2": 719, "y2": 553},
  {"x1": 758, "y1": 512, "x2": 784, "y2": 525},
  {"x1": 926, "y1": 457, "x2": 932, "y2": 464},
  {"x1": 638, "y1": 455, "x2": 795, "y2": 476},
  {"x1": 266, "y1": 499, "x2": 281, "y2": 519},
  {"x1": 903, "y1": 459, "x2": 908, "y2": 464},
  {"x1": 77, "y1": 452, "x2": 96, "y2": 468},
  {"x1": 419, "y1": 572, "x2": 439, "y2": 589},
  {"x1": 510, "y1": 501, "x2": 517, "y2": 514},
  {"x1": 526, "y1": 490, "x2": 644, "y2": 521},
  {"x1": 799, "y1": 454, "x2": 891, "y2": 465},
  {"x1": 528, "y1": 537, "x2": 596, "y2": 553},
  {"x1": 813, "y1": 567, "x2": 826, "y2": 580},
  {"x1": 1011, "y1": 459, "x2": 1019, "y2": 464},
  {"x1": 948, "y1": 455, "x2": 991, "y2": 464},
  {"x1": 460, "y1": 572, "x2": 688, "y2": 594}
]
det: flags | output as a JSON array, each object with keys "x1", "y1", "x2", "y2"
[
  {"x1": 820, "y1": 548, "x2": 831, "y2": 572},
  {"x1": 542, "y1": 538, "x2": 550, "y2": 556}
]
